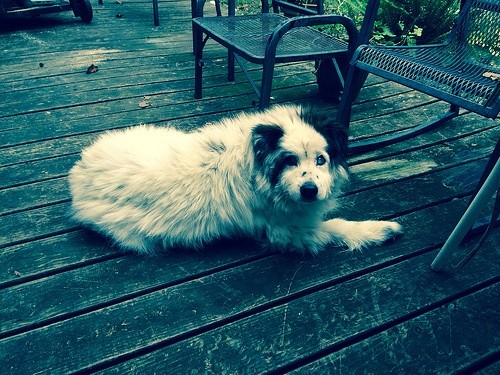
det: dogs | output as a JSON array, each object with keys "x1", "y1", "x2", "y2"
[{"x1": 66, "y1": 103, "x2": 404, "y2": 255}]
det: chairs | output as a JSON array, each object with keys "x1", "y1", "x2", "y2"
[
  {"x1": 333, "y1": 1, "x2": 500, "y2": 275},
  {"x1": 188, "y1": 0, "x2": 381, "y2": 116}
]
